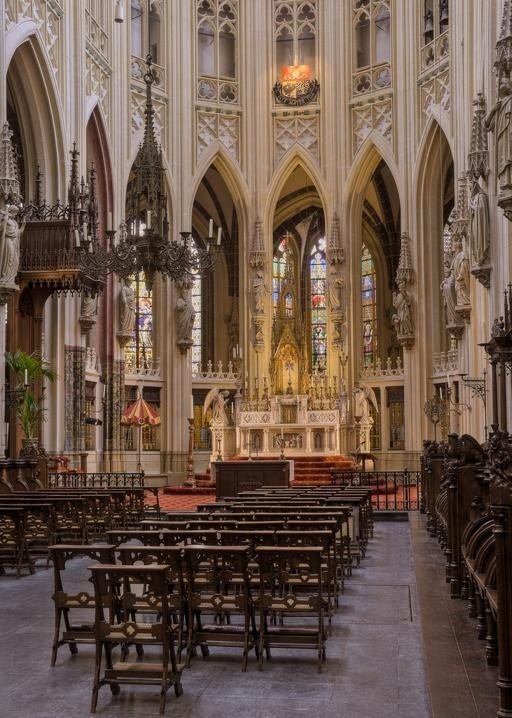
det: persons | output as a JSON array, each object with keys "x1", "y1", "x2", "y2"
[
  {"x1": 177, "y1": 289, "x2": 195, "y2": 342},
  {"x1": 253, "y1": 270, "x2": 270, "y2": 313},
  {"x1": 356, "y1": 388, "x2": 370, "y2": 417},
  {"x1": 440, "y1": 269, "x2": 464, "y2": 324},
  {"x1": 213, "y1": 390, "x2": 230, "y2": 426},
  {"x1": 121, "y1": 278, "x2": 135, "y2": 333},
  {"x1": 482, "y1": 77, "x2": 512, "y2": 190},
  {"x1": 468, "y1": 181, "x2": 489, "y2": 267},
  {"x1": 392, "y1": 284, "x2": 413, "y2": 335},
  {"x1": 328, "y1": 272, "x2": 344, "y2": 308},
  {"x1": 450, "y1": 242, "x2": 470, "y2": 305},
  {"x1": 0, "y1": 204, "x2": 26, "y2": 291}
]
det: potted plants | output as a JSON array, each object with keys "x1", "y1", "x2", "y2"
[{"x1": 2, "y1": 347, "x2": 61, "y2": 452}]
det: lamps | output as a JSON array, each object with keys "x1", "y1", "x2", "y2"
[{"x1": 68, "y1": 1, "x2": 224, "y2": 299}]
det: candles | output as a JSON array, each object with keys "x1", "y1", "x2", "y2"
[
  {"x1": 460, "y1": 356, "x2": 465, "y2": 372},
  {"x1": 280, "y1": 427, "x2": 284, "y2": 439},
  {"x1": 446, "y1": 374, "x2": 450, "y2": 388},
  {"x1": 24, "y1": 368, "x2": 28, "y2": 385},
  {"x1": 439, "y1": 386, "x2": 443, "y2": 400},
  {"x1": 189, "y1": 394, "x2": 194, "y2": 419}
]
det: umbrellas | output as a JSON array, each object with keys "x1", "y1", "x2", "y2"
[{"x1": 121, "y1": 394, "x2": 161, "y2": 464}]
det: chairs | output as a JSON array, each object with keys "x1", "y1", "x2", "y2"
[{"x1": 0, "y1": 486, "x2": 376, "y2": 714}]
